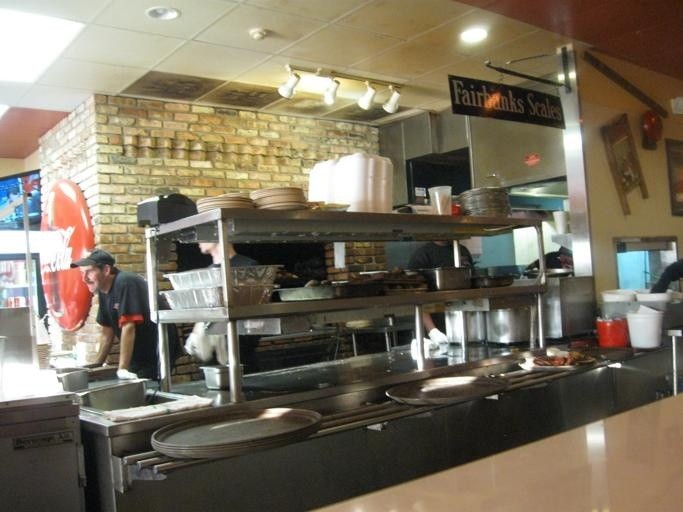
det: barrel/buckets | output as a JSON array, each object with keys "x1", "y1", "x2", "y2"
[
  {"x1": 597, "y1": 317, "x2": 629, "y2": 351},
  {"x1": 627, "y1": 312, "x2": 665, "y2": 351}
]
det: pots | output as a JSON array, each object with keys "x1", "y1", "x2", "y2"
[
  {"x1": 335, "y1": 283, "x2": 383, "y2": 298},
  {"x1": 474, "y1": 275, "x2": 514, "y2": 288},
  {"x1": 444, "y1": 306, "x2": 531, "y2": 345},
  {"x1": 274, "y1": 285, "x2": 336, "y2": 301}
]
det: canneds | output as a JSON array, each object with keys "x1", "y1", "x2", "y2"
[{"x1": 7, "y1": 296, "x2": 29, "y2": 308}]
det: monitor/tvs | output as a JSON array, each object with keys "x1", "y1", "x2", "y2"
[
  {"x1": 0, "y1": 169, "x2": 41, "y2": 231},
  {"x1": 0, "y1": 307, "x2": 33, "y2": 366}
]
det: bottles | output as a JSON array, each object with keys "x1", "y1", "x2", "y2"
[{"x1": 46, "y1": 191, "x2": 63, "y2": 315}]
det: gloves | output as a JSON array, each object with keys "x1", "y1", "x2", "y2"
[
  {"x1": 116, "y1": 369, "x2": 138, "y2": 380},
  {"x1": 410, "y1": 327, "x2": 449, "y2": 360}
]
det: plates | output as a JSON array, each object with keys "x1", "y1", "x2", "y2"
[
  {"x1": 195, "y1": 193, "x2": 253, "y2": 212},
  {"x1": 457, "y1": 186, "x2": 512, "y2": 218},
  {"x1": 250, "y1": 187, "x2": 308, "y2": 209}
]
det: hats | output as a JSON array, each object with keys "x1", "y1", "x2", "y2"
[{"x1": 70, "y1": 250, "x2": 115, "y2": 268}]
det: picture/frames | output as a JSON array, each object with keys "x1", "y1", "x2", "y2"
[{"x1": 664, "y1": 137, "x2": 683, "y2": 216}]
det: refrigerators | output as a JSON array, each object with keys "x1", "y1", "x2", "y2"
[{"x1": 0, "y1": 168, "x2": 41, "y2": 316}]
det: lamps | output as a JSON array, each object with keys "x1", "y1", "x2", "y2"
[{"x1": 279, "y1": 63, "x2": 406, "y2": 115}]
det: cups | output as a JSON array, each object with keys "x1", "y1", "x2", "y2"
[{"x1": 428, "y1": 186, "x2": 452, "y2": 215}]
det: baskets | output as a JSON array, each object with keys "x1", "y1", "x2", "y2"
[{"x1": 158, "y1": 264, "x2": 284, "y2": 310}]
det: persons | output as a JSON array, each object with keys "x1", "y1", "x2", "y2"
[
  {"x1": 69, "y1": 249, "x2": 183, "y2": 384},
  {"x1": 524, "y1": 245, "x2": 572, "y2": 276},
  {"x1": 408, "y1": 239, "x2": 475, "y2": 345},
  {"x1": 196, "y1": 241, "x2": 281, "y2": 374}
]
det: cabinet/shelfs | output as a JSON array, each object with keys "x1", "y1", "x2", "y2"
[
  {"x1": 0, "y1": 252, "x2": 40, "y2": 338},
  {"x1": 143, "y1": 204, "x2": 554, "y2": 404}
]
